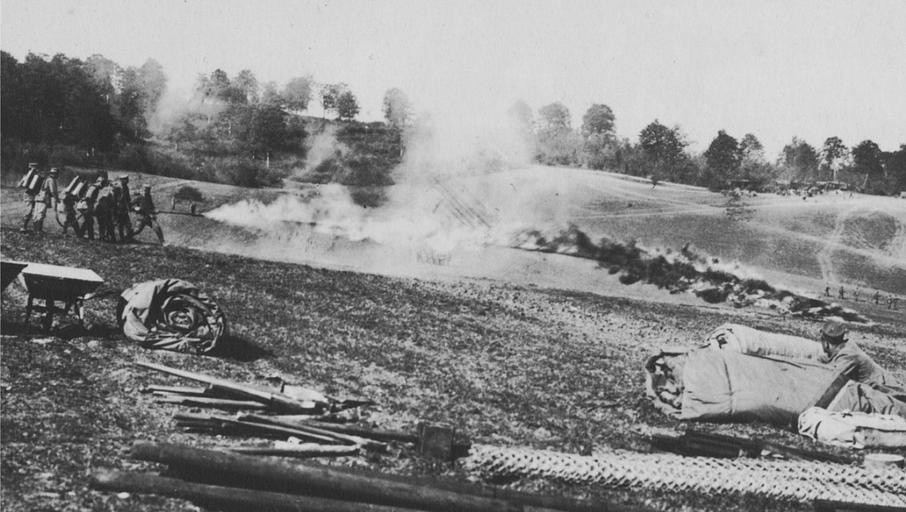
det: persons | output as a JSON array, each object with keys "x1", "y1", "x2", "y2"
[
  {"x1": 18, "y1": 162, "x2": 166, "y2": 246},
  {"x1": 823, "y1": 281, "x2": 900, "y2": 313},
  {"x1": 811, "y1": 321, "x2": 903, "y2": 413}
]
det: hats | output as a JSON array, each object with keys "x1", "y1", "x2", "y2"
[
  {"x1": 29, "y1": 162, "x2": 38, "y2": 168},
  {"x1": 822, "y1": 314, "x2": 847, "y2": 338},
  {"x1": 120, "y1": 173, "x2": 129, "y2": 182}
]
